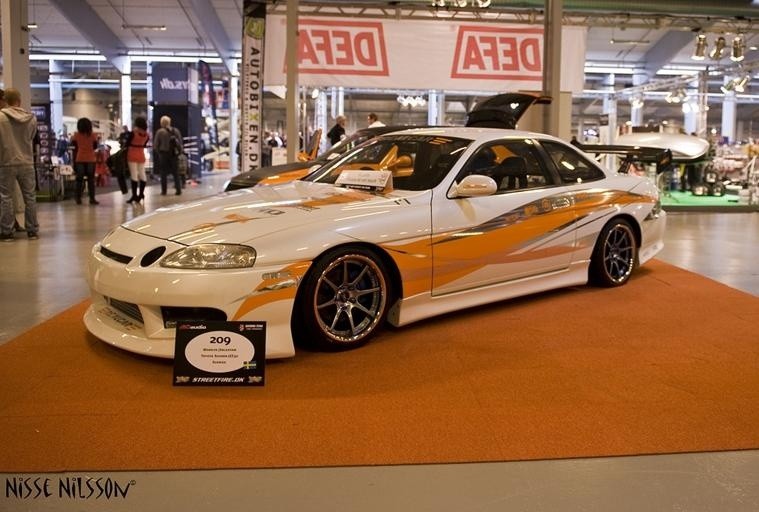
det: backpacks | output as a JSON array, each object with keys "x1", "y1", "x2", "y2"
[{"x1": 167, "y1": 127, "x2": 184, "y2": 157}]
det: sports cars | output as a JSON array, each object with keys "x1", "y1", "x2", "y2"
[{"x1": 81, "y1": 90, "x2": 711, "y2": 371}]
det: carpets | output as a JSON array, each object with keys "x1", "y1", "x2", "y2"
[{"x1": 1, "y1": 259, "x2": 759, "y2": 473}]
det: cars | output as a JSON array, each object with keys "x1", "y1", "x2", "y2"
[{"x1": 201, "y1": 146, "x2": 233, "y2": 171}]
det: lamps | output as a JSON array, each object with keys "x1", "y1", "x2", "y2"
[
  {"x1": 626, "y1": 27, "x2": 750, "y2": 109},
  {"x1": 431, "y1": 0, "x2": 491, "y2": 8},
  {"x1": 395, "y1": 93, "x2": 426, "y2": 107}
]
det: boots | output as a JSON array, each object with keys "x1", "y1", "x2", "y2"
[
  {"x1": 138, "y1": 179, "x2": 147, "y2": 202},
  {"x1": 126, "y1": 179, "x2": 139, "y2": 203}
]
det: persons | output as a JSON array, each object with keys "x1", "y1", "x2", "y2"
[
  {"x1": 0, "y1": 86, "x2": 40, "y2": 242},
  {"x1": 326, "y1": 115, "x2": 347, "y2": 146},
  {"x1": 69, "y1": 115, "x2": 99, "y2": 206},
  {"x1": 126, "y1": 117, "x2": 150, "y2": 204},
  {"x1": 367, "y1": 113, "x2": 389, "y2": 129},
  {"x1": 119, "y1": 124, "x2": 131, "y2": 143},
  {"x1": 152, "y1": 115, "x2": 183, "y2": 196},
  {"x1": 0, "y1": 90, "x2": 25, "y2": 233},
  {"x1": 236, "y1": 130, "x2": 303, "y2": 170}
]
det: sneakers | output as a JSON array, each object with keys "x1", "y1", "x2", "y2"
[
  {"x1": 90, "y1": 199, "x2": 99, "y2": 204},
  {"x1": 28, "y1": 231, "x2": 39, "y2": 240},
  {"x1": 1, "y1": 232, "x2": 16, "y2": 242},
  {"x1": 74, "y1": 197, "x2": 82, "y2": 204}
]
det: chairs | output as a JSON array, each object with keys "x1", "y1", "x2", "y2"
[{"x1": 413, "y1": 146, "x2": 527, "y2": 191}]
